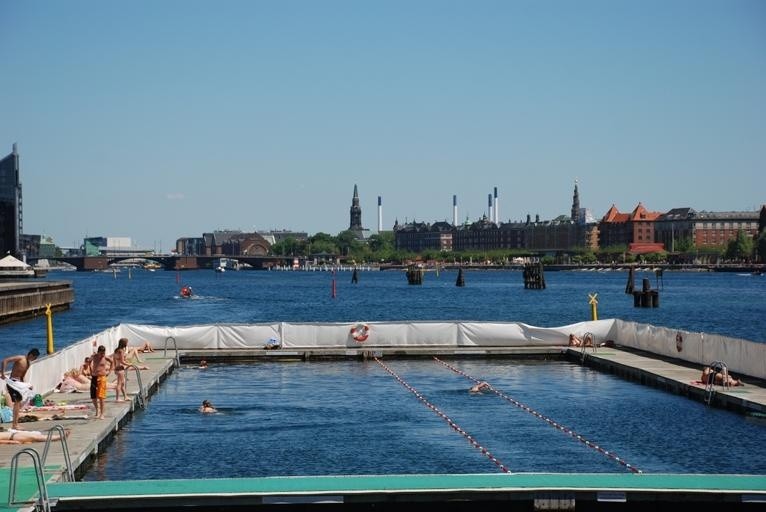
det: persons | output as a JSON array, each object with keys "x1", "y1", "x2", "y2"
[
  {"x1": 181, "y1": 286, "x2": 193, "y2": 297},
  {"x1": 701, "y1": 366, "x2": 744, "y2": 387},
  {"x1": 470, "y1": 382, "x2": 489, "y2": 393},
  {"x1": 198, "y1": 360, "x2": 209, "y2": 369},
  {"x1": 0, "y1": 338, "x2": 154, "y2": 443},
  {"x1": 203, "y1": 399, "x2": 218, "y2": 412},
  {"x1": 568, "y1": 334, "x2": 594, "y2": 348}
]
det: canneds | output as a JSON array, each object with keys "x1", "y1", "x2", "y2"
[
  {"x1": 676, "y1": 334, "x2": 683, "y2": 352},
  {"x1": 351, "y1": 324, "x2": 369, "y2": 341}
]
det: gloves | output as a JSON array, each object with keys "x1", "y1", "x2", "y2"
[
  {"x1": 172, "y1": 293, "x2": 204, "y2": 301},
  {"x1": 751, "y1": 272, "x2": 762, "y2": 276},
  {"x1": 214, "y1": 266, "x2": 227, "y2": 273},
  {"x1": 147, "y1": 267, "x2": 155, "y2": 273}
]
